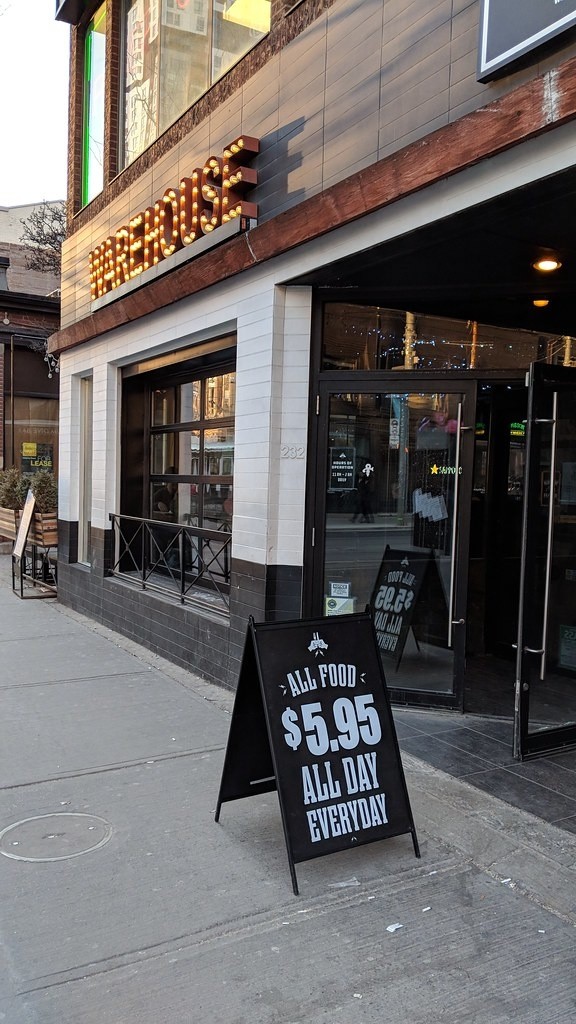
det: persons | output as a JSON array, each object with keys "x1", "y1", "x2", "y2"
[
  {"x1": 152, "y1": 466, "x2": 178, "y2": 514},
  {"x1": 348, "y1": 461, "x2": 375, "y2": 524}
]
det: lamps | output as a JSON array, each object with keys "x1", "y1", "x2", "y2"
[{"x1": 531, "y1": 254, "x2": 562, "y2": 272}]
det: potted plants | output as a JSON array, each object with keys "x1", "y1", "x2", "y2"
[{"x1": 0, "y1": 465, "x2": 58, "y2": 545}]
